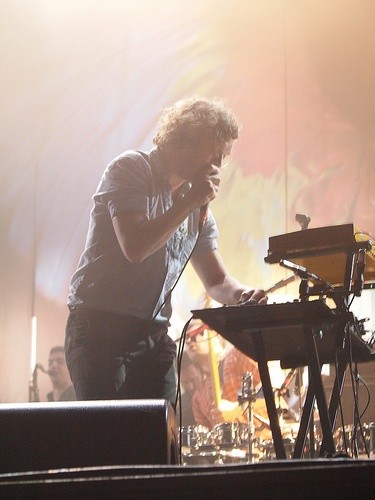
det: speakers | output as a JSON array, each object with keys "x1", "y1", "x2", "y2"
[{"x1": 0, "y1": 399, "x2": 180, "y2": 473}]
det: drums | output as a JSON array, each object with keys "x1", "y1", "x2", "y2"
[
  {"x1": 262, "y1": 437, "x2": 312, "y2": 460},
  {"x1": 212, "y1": 422, "x2": 255, "y2": 449},
  {"x1": 336, "y1": 422, "x2": 375, "y2": 455},
  {"x1": 178, "y1": 424, "x2": 211, "y2": 456}
]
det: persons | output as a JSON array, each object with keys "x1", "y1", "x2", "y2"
[
  {"x1": 216, "y1": 330, "x2": 238, "y2": 391},
  {"x1": 176, "y1": 349, "x2": 205, "y2": 426},
  {"x1": 47, "y1": 346, "x2": 79, "y2": 401},
  {"x1": 186, "y1": 318, "x2": 289, "y2": 439},
  {"x1": 65, "y1": 100, "x2": 268, "y2": 402}
]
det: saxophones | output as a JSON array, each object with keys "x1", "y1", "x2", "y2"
[{"x1": 28, "y1": 364, "x2": 54, "y2": 402}]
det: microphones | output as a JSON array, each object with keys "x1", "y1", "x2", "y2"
[
  {"x1": 353, "y1": 249, "x2": 365, "y2": 296},
  {"x1": 196, "y1": 172, "x2": 217, "y2": 232}
]
